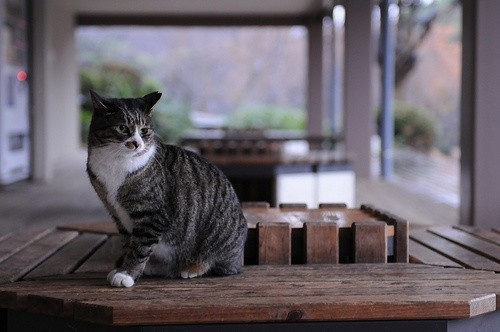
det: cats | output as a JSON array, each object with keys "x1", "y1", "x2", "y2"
[{"x1": 85, "y1": 88, "x2": 249, "y2": 288}]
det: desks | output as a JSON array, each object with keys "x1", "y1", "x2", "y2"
[
  {"x1": 180, "y1": 128, "x2": 356, "y2": 207},
  {"x1": 1, "y1": 202, "x2": 500, "y2": 332}
]
